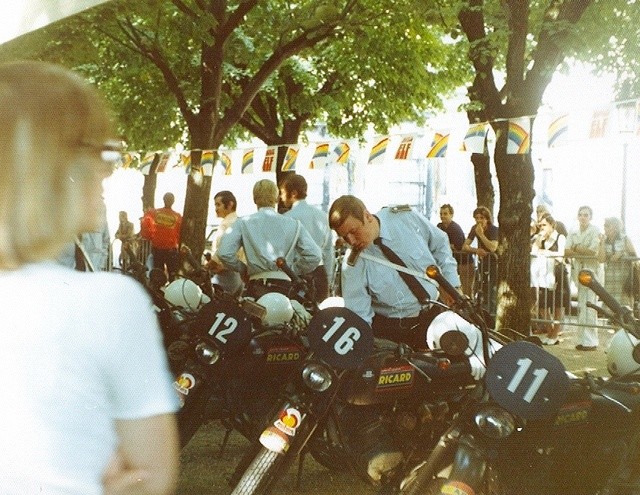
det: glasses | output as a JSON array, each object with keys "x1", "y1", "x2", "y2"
[
  {"x1": 77, "y1": 136, "x2": 131, "y2": 161},
  {"x1": 538, "y1": 224, "x2": 547, "y2": 227},
  {"x1": 578, "y1": 214, "x2": 589, "y2": 218}
]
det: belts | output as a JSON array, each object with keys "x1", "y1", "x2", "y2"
[{"x1": 397, "y1": 318, "x2": 414, "y2": 330}]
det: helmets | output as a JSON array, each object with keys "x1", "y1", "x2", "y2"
[
  {"x1": 163, "y1": 278, "x2": 203, "y2": 324},
  {"x1": 607, "y1": 327, "x2": 640, "y2": 377},
  {"x1": 252, "y1": 292, "x2": 293, "y2": 329},
  {"x1": 318, "y1": 296, "x2": 344, "y2": 311},
  {"x1": 426, "y1": 309, "x2": 478, "y2": 357}
]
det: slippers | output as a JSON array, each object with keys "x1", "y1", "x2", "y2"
[
  {"x1": 540, "y1": 337, "x2": 550, "y2": 345},
  {"x1": 546, "y1": 337, "x2": 559, "y2": 345}
]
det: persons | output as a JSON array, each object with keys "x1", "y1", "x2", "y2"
[
  {"x1": 328, "y1": 195, "x2": 463, "y2": 352},
  {"x1": 529, "y1": 213, "x2": 567, "y2": 339},
  {"x1": 536, "y1": 205, "x2": 568, "y2": 331},
  {"x1": 141, "y1": 192, "x2": 183, "y2": 283},
  {"x1": 54, "y1": 219, "x2": 111, "y2": 272},
  {"x1": 204, "y1": 191, "x2": 245, "y2": 301},
  {"x1": 564, "y1": 206, "x2": 605, "y2": 350},
  {"x1": 215, "y1": 179, "x2": 323, "y2": 302},
  {"x1": 598, "y1": 217, "x2": 636, "y2": 316},
  {"x1": 0, "y1": 59, "x2": 183, "y2": 495},
  {"x1": 436, "y1": 205, "x2": 475, "y2": 299},
  {"x1": 278, "y1": 175, "x2": 336, "y2": 302},
  {"x1": 462, "y1": 206, "x2": 499, "y2": 312},
  {"x1": 115, "y1": 211, "x2": 134, "y2": 266}
]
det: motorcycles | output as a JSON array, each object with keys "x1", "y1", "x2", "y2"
[
  {"x1": 167, "y1": 243, "x2": 399, "y2": 470},
  {"x1": 379, "y1": 265, "x2": 640, "y2": 495},
  {"x1": 227, "y1": 257, "x2": 544, "y2": 495}
]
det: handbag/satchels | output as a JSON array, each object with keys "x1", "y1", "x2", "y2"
[
  {"x1": 625, "y1": 271, "x2": 640, "y2": 295},
  {"x1": 556, "y1": 262, "x2": 568, "y2": 281}
]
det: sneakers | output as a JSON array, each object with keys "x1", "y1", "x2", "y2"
[
  {"x1": 581, "y1": 346, "x2": 596, "y2": 350},
  {"x1": 576, "y1": 345, "x2": 582, "y2": 350}
]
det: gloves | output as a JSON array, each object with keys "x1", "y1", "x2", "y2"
[{"x1": 468, "y1": 324, "x2": 487, "y2": 381}]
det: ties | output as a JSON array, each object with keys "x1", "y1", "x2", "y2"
[{"x1": 372, "y1": 237, "x2": 430, "y2": 304}]
